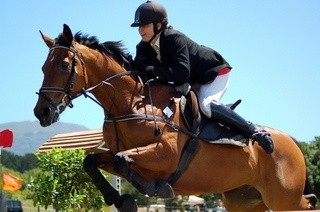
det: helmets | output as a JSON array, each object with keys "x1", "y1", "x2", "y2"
[{"x1": 130, "y1": 1, "x2": 168, "y2": 27}]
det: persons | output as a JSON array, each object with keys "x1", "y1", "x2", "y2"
[{"x1": 130, "y1": 0, "x2": 274, "y2": 155}]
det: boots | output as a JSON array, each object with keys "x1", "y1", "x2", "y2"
[{"x1": 208, "y1": 101, "x2": 274, "y2": 155}]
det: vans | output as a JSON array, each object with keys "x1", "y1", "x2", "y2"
[{"x1": 6, "y1": 199, "x2": 23, "y2": 212}]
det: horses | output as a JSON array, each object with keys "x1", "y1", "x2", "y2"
[{"x1": 33, "y1": 23, "x2": 318, "y2": 212}]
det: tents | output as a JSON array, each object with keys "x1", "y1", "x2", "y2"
[{"x1": 158, "y1": 194, "x2": 209, "y2": 212}]
